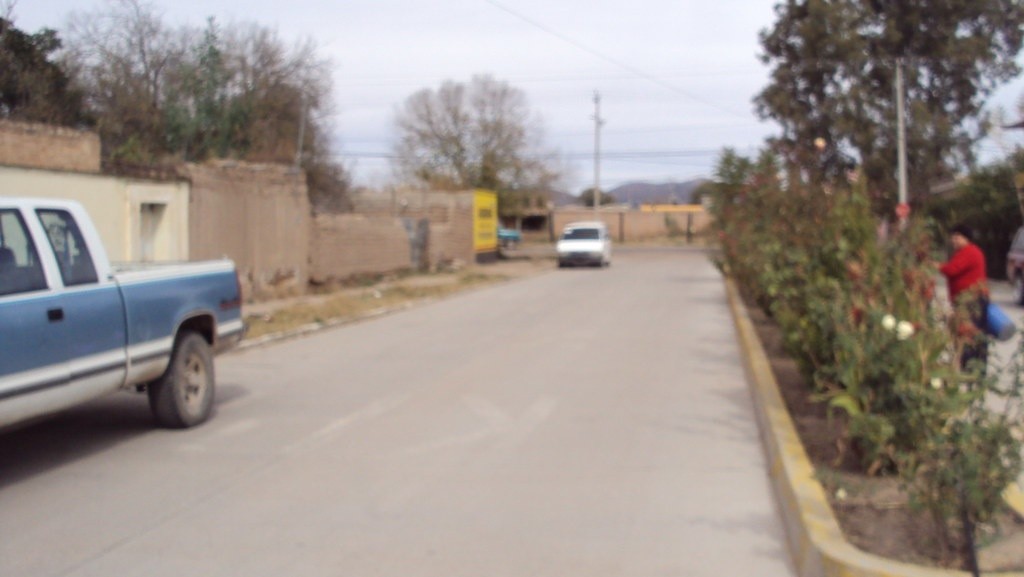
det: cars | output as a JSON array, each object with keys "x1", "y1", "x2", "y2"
[
  {"x1": 496, "y1": 221, "x2": 522, "y2": 256},
  {"x1": 555, "y1": 221, "x2": 612, "y2": 266}
]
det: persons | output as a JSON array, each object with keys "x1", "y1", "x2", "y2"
[
  {"x1": 1007, "y1": 225, "x2": 1024, "y2": 306},
  {"x1": 924, "y1": 221, "x2": 989, "y2": 377}
]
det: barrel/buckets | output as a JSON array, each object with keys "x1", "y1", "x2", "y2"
[{"x1": 987, "y1": 304, "x2": 1016, "y2": 342}]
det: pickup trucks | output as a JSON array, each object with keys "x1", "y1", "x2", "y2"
[{"x1": 0, "y1": 198, "x2": 242, "y2": 438}]
